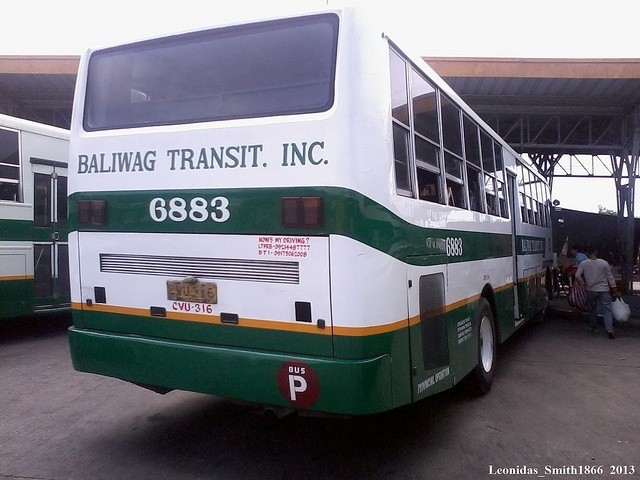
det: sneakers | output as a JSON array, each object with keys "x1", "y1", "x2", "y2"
[{"x1": 608, "y1": 329, "x2": 616, "y2": 339}]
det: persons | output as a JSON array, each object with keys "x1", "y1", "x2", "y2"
[
  {"x1": 574, "y1": 245, "x2": 622, "y2": 340},
  {"x1": 570, "y1": 241, "x2": 588, "y2": 269}
]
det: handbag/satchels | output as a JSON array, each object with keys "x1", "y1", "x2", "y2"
[{"x1": 610, "y1": 297, "x2": 631, "y2": 322}]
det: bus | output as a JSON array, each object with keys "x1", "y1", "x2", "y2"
[
  {"x1": 66, "y1": 11, "x2": 554, "y2": 417},
  {"x1": 0, "y1": 114, "x2": 72, "y2": 321}
]
